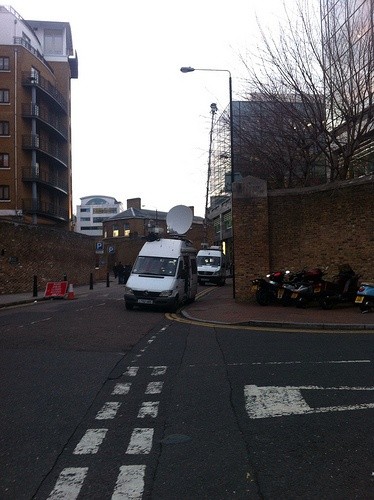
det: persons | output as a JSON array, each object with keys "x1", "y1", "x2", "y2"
[
  {"x1": 113, "y1": 262, "x2": 117, "y2": 279},
  {"x1": 117, "y1": 261, "x2": 132, "y2": 284}
]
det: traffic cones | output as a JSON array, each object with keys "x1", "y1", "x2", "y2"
[{"x1": 67, "y1": 284, "x2": 75, "y2": 300}]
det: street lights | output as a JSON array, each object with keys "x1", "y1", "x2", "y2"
[{"x1": 180, "y1": 66, "x2": 235, "y2": 298}]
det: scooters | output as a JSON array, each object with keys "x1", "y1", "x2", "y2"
[{"x1": 252, "y1": 266, "x2": 374, "y2": 313}]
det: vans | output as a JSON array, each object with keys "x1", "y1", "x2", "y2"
[
  {"x1": 124, "y1": 235, "x2": 197, "y2": 313},
  {"x1": 196, "y1": 250, "x2": 226, "y2": 286}
]
